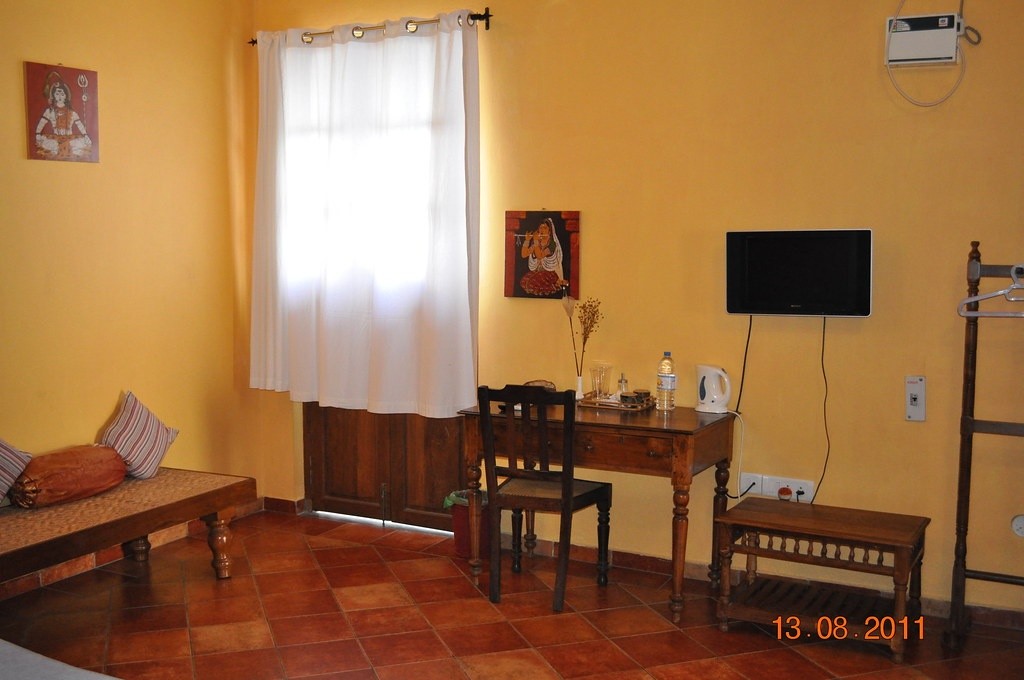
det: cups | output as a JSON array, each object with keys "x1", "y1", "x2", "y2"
[
  {"x1": 591, "y1": 365, "x2": 602, "y2": 398},
  {"x1": 634, "y1": 389, "x2": 651, "y2": 403},
  {"x1": 620, "y1": 392, "x2": 639, "y2": 406},
  {"x1": 602, "y1": 363, "x2": 610, "y2": 399}
]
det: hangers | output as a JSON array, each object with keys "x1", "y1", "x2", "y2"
[{"x1": 958, "y1": 265, "x2": 1024, "y2": 319}]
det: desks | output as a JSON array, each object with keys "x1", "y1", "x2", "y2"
[
  {"x1": 712, "y1": 497, "x2": 931, "y2": 665},
  {"x1": 457, "y1": 398, "x2": 742, "y2": 622}
]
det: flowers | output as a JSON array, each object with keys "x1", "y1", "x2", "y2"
[{"x1": 558, "y1": 295, "x2": 605, "y2": 375}]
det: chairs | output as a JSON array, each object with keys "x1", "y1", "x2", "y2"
[{"x1": 477, "y1": 383, "x2": 612, "y2": 617}]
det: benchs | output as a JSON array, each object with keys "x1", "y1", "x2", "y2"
[{"x1": 0, "y1": 467, "x2": 258, "y2": 580}]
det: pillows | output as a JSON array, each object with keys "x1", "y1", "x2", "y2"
[
  {"x1": 0, "y1": 440, "x2": 33, "y2": 504},
  {"x1": 102, "y1": 391, "x2": 180, "y2": 482},
  {"x1": 6, "y1": 446, "x2": 125, "y2": 510}
]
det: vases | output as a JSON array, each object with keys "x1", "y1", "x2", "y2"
[{"x1": 575, "y1": 377, "x2": 584, "y2": 401}]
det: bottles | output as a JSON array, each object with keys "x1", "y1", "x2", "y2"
[
  {"x1": 656, "y1": 352, "x2": 676, "y2": 410},
  {"x1": 616, "y1": 374, "x2": 630, "y2": 400}
]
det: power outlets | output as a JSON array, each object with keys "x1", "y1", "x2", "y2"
[
  {"x1": 782, "y1": 476, "x2": 814, "y2": 503},
  {"x1": 739, "y1": 472, "x2": 762, "y2": 495}
]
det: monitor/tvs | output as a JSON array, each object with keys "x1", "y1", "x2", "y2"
[{"x1": 726, "y1": 229, "x2": 873, "y2": 317}]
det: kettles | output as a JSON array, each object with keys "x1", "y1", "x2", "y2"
[{"x1": 694, "y1": 365, "x2": 732, "y2": 413}]
